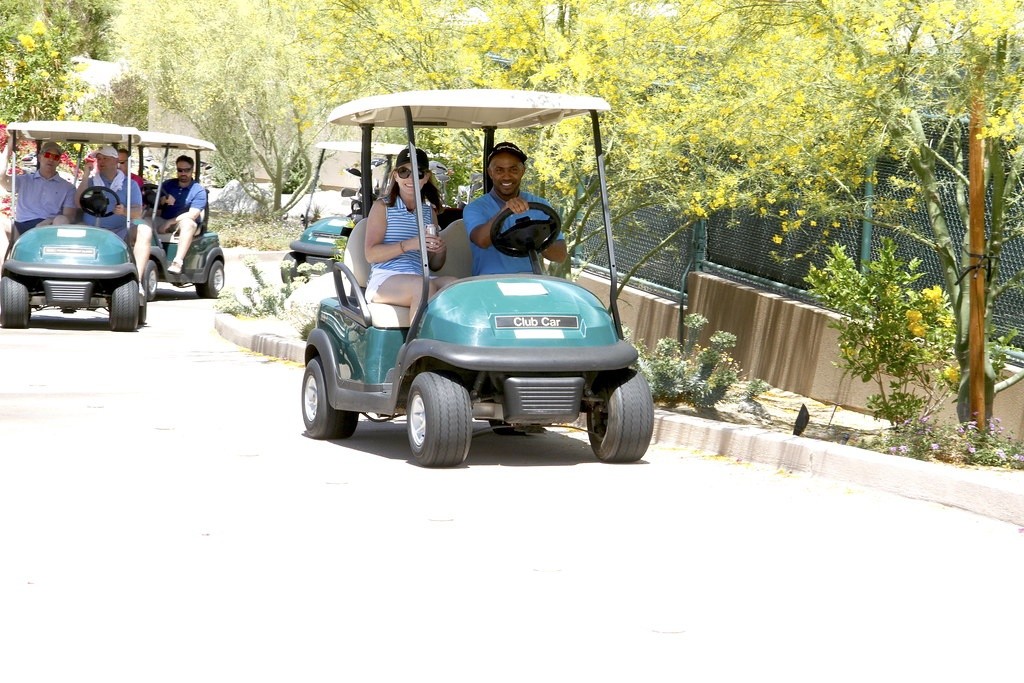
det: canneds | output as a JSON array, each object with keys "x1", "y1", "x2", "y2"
[{"x1": 425, "y1": 224, "x2": 439, "y2": 245}]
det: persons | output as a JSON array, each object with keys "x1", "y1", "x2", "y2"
[
  {"x1": 73, "y1": 145, "x2": 154, "y2": 298},
  {"x1": 144, "y1": 155, "x2": 207, "y2": 272},
  {"x1": 460, "y1": 140, "x2": 568, "y2": 276},
  {"x1": 364, "y1": 146, "x2": 463, "y2": 329},
  {"x1": 115, "y1": 147, "x2": 145, "y2": 220},
  {"x1": 0, "y1": 132, "x2": 76, "y2": 249}
]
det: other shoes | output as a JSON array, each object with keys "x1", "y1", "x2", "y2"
[{"x1": 167, "y1": 260, "x2": 182, "y2": 275}]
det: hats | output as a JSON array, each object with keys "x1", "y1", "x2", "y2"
[
  {"x1": 40, "y1": 142, "x2": 65, "y2": 156},
  {"x1": 397, "y1": 149, "x2": 429, "y2": 170},
  {"x1": 488, "y1": 141, "x2": 527, "y2": 166},
  {"x1": 91, "y1": 147, "x2": 118, "y2": 159}
]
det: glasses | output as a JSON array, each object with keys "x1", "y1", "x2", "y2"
[
  {"x1": 394, "y1": 167, "x2": 424, "y2": 181},
  {"x1": 117, "y1": 159, "x2": 127, "y2": 165},
  {"x1": 42, "y1": 151, "x2": 61, "y2": 162},
  {"x1": 177, "y1": 165, "x2": 194, "y2": 173}
]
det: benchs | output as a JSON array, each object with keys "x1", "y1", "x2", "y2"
[
  {"x1": 343, "y1": 216, "x2": 477, "y2": 329},
  {"x1": 128, "y1": 203, "x2": 203, "y2": 245}
]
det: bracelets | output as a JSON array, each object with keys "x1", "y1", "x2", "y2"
[
  {"x1": 46, "y1": 217, "x2": 52, "y2": 225},
  {"x1": 400, "y1": 240, "x2": 406, "y2": 254}
]
